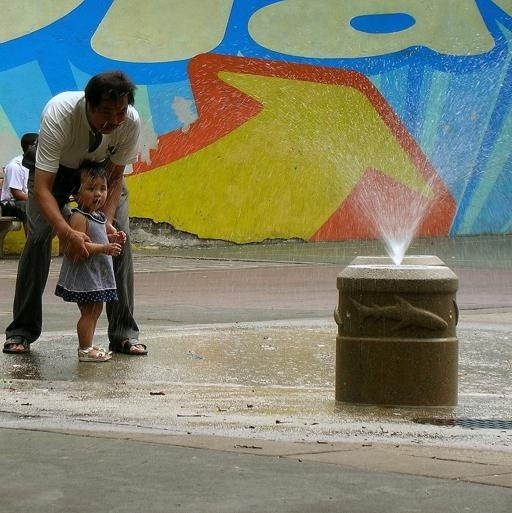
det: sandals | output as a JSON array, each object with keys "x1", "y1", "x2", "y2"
[
  {"x1": 77, "y1": 345, "x2": 113, "y2": 362},
  {"x1": 109, "y1": 339, "x2": 147, "y2": 355},
  {"x1": 2, "y1": 337, "x2": 31, "y2": 354}
]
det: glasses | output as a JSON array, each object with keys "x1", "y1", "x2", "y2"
[{"x1": 94, "y1": 119, "x2": 127, "y2": 131}]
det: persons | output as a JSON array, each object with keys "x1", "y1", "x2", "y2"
[
  {"x1": 0, "y1": 133, "x2": 38, "y2": 225},
  {"x1": 3, "y1": 71, "x2": 148, "y2": 355},
  {"x1": 55, "y1": 159, "x2": 126, "y2": 362}
]
